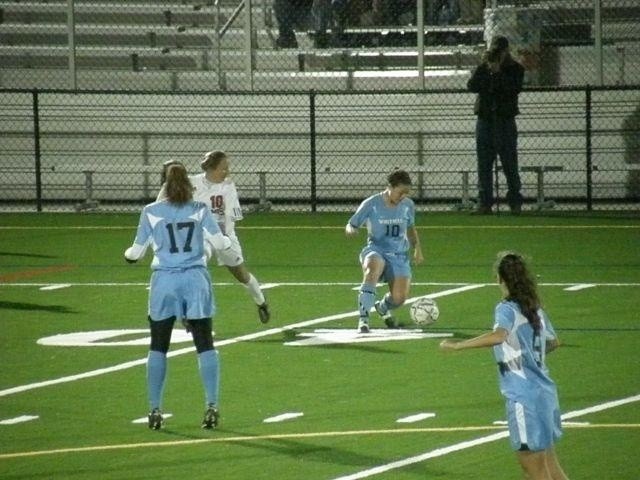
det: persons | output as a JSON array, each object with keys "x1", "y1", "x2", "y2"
[
  {"x1": 344, "y1": 169, "x2": 424, "y2": 333},
  {"x1": 123, "y1": 159, "x2": 231, "y2": 431},
  {"x1": 271, "y1": 1, "x2": 488, "y2": 49},
  {"x1": 440, "y1": 253, "x2": 571, "y2": 480},
  {"x1": 466, "y1": 35, "x2": 525, "y2": 217},
  {"x1": 183, "y1": 150, "x2": 270, "y2": 331}
]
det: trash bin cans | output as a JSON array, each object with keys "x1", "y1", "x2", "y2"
[{"x1": 484, "y1": 6, "x2": 543, "y2": 88}]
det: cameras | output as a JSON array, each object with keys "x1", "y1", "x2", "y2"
[{"x1": 486, "y1": 49, "x2": 501, "y2": 64}]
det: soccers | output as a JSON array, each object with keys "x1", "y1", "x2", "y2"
[{"x1": 410, "y1": 297, "x2": 440, "y2": 327}]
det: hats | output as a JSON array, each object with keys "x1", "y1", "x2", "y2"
[{"x1": 489, "y1": 35, "x2": 509, "y2": 53}]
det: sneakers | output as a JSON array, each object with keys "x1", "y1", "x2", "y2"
[
  {"x1": 357, "y1": 316, "x2": 371, "y2": 334},
  {"x1": 508, "y1": 199, "x2": 522, "y2": 216},
  {"x1": 469, "y1": 201, "x2": 493, "y2": 216},
  {"x1": 200, "y1": 405, "x2": 220, "y2": 430},
  {"x1": 148, "y1": 407, "x2": 163, "y2": 431},
  {"x1": 255, "y1": 298, "x2": 270, "y2": 324},
  {"x1": 374, "y1": 300, "x2": 396, "y2": 327},
  {"x1": 272, "y1": 11, "x2": 482, "y2": 48}
]
